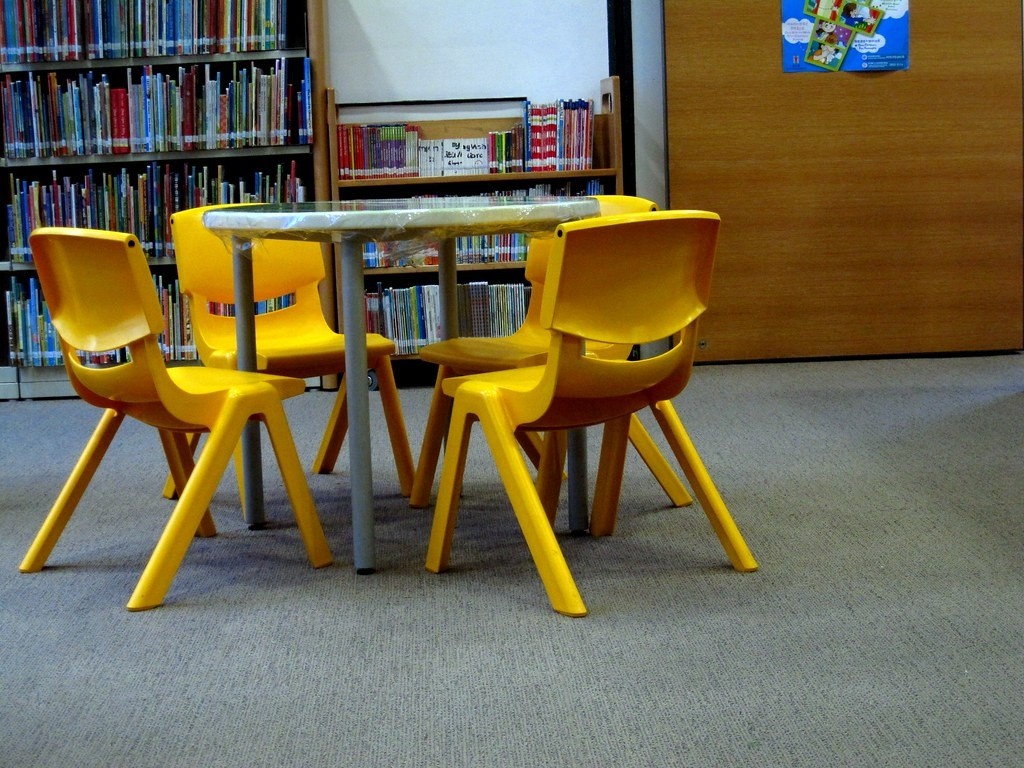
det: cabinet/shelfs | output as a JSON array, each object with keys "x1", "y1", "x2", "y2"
[
  {"x1": 325, "y1": 76, "x2": 627, "y2": 391},
  {"x1": 0, "y1": 0, "x2": 338, "y2": 398}
]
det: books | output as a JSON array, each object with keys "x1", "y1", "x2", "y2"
[
  {"x1": 0, "y1": 0, "x2": 314, "y2": 368},
  {"x1": 337, "y1": 97, "x2": 605, "y2": 357}
]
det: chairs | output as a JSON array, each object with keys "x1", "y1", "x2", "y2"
[
  {"x1": 408, "y1": 195, "x2": 693, "y2": 531},
  {"x1": 425, "y1": 210, "x2": 758, "y2": 616},
  {"x1": 18, "y1": 226, "x2": 334, "y2": 611},
  {"x1": 162, "y1": 203, "x2": 414, "y2": 524}
]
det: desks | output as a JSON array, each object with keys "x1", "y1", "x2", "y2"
[{"x1": 203, "y1": 196, "x2": 601, "y2": 576}]
